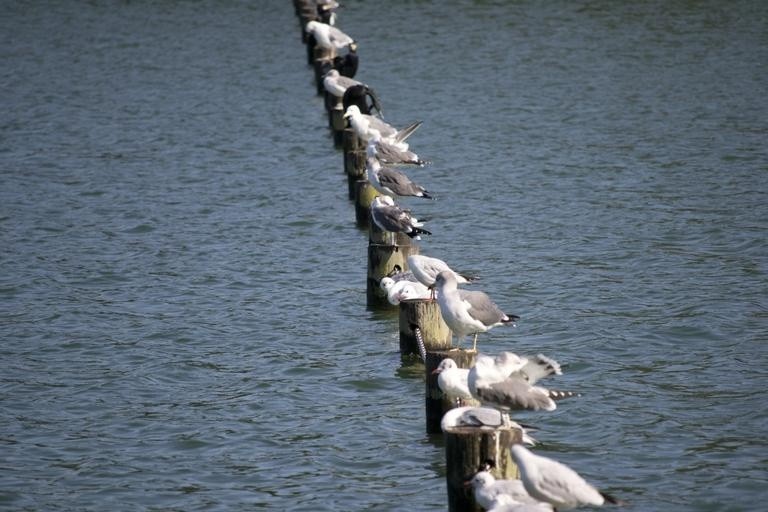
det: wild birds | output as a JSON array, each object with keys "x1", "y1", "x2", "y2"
[
  {"x1": 380, "y1": 254, "x2": 475, "y2": 306},
  {"x1": 431, "y1": 350, "x2": 582, "y2": 449},
  {"x1": 427, "y1": 270, "x2": 521, "y2": 353},
  {"x1": 370, "y1": 195, "x2": 434, "y2": 246},
  {"x1": 362, "y1": 156, "x2": 438, "y2": 202},
  {"x1": 306, "y1": 0, "x2": 372, "y2": 98},
  {"x1": 341, "y1": 84, "x2": 434, "y2": 168},
  {"x1": 464, "y1": 443, "x2": 632, "y2": 512}
]
